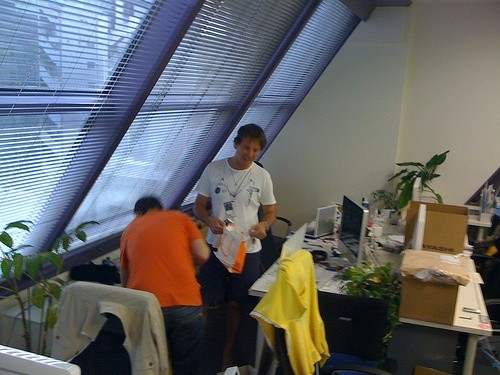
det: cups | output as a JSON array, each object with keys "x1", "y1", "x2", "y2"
[
  {"x1": 362, "y1": 201, "x2": 369, "y2": 209},
  {"x1": 373, "y1": 223, "x2": 383, "y2": 238}
]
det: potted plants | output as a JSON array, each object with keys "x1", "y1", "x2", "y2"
[{"x1": 370, "y1": 188, "x2": 397, "y2": 221}]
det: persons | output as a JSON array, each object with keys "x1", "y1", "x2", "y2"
[
  {"x1": 119, "y1": 196, "x2": 210, "y2": 375},
  {"x1": 194, "y1": 124, "x2": 278, "y2": 375}
]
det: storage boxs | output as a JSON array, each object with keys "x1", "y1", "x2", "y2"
[
  {"x1": 402, "y1": 200, "x2": 470, "y2": 254},
  {"x1": 399, "y1": 248, "x2": 470, "y2": 325}
]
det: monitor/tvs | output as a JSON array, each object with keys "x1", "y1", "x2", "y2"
[
  {"x1": 337, "y1": 194, "x2": 370, "y2": 269},
  {"x1": 0, "y1": 344, "x2": 81, "y2": 375},
  {"x1": 407, "y1": 178, "x2": 427, "y2": 251}
]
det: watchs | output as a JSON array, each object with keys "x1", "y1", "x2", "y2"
[{"x1": 262, "y1": 220, "x2": 269, "y2": 231}]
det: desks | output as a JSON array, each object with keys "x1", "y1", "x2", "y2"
[{"x1": 247, "y1": 218, "x2": 492, "y2": 375}]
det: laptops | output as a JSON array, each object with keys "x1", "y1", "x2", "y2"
[{"x1": 291, "y1": 205, "x2": 338, "y2": 239}]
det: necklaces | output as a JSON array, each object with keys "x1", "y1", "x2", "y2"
[{"x1": 229, "y1": 159, "x2": 252, "y2": 186}]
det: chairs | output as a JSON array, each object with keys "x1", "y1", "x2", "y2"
[
  {"x1": 250, "y1": 248, "x2": 393, "y2": 375},
  {"x1": 50, "y1": 281, "x2": 178, "y2": 375}
]
